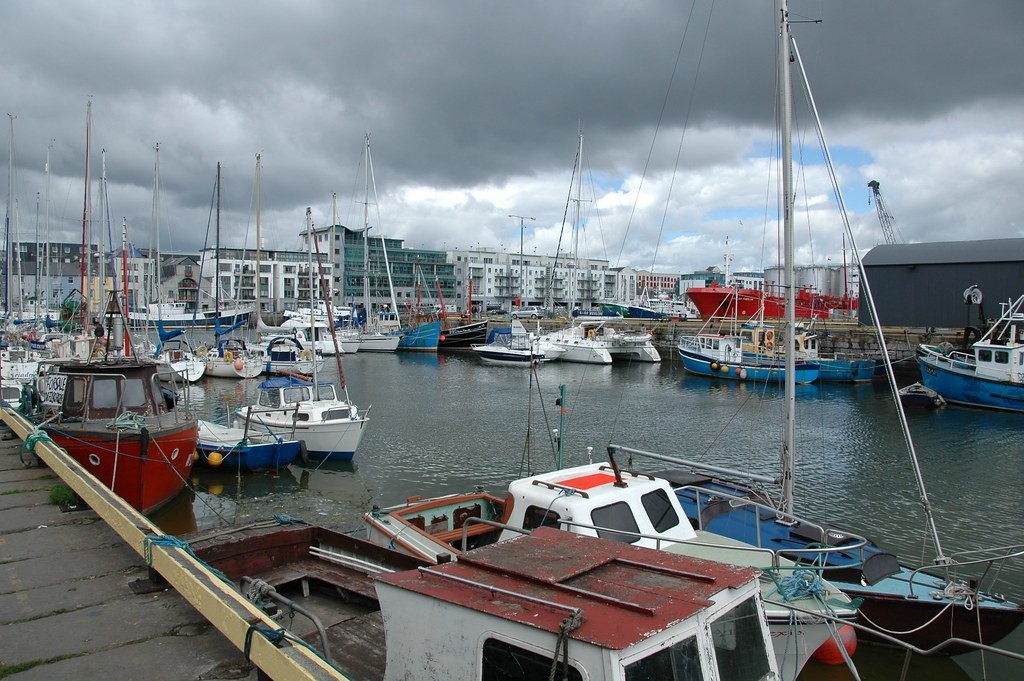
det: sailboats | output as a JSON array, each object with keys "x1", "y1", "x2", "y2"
[{"x1": 3, "y1": 0, "x2": 1024, "y2": 681}]
